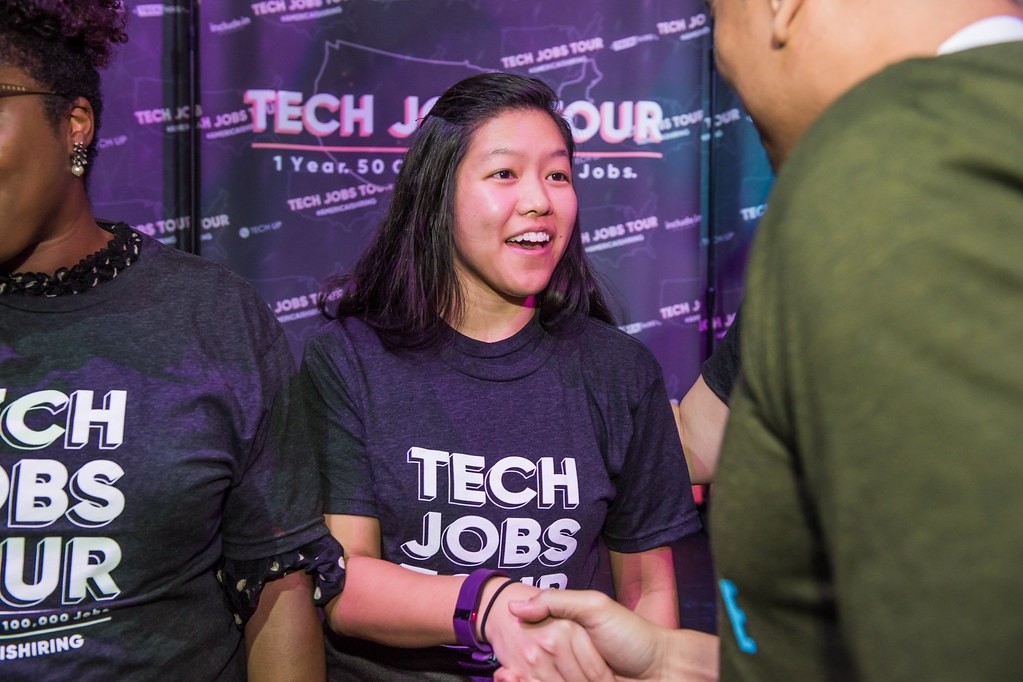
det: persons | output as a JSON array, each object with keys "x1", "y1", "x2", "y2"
[
  {"x1": 1, "y1": 0, "x2": 346, "y2": 682},
  {"x1": 670, "y1": 305, "x2": 738, "y2": 484},
  {"x1": 298, "y1": 71, "x2": 702, "y2": 682},
  {"x1": 494, "y1": 0, "x2": 1023, "y2": 681}
]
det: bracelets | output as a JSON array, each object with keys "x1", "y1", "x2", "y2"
[
  {"x1": 440, "y1": 568, "x2": 510, "y2": 667},
  {"x1": 481, "y1": 578, "x2": 522, "y2": 643}
]
